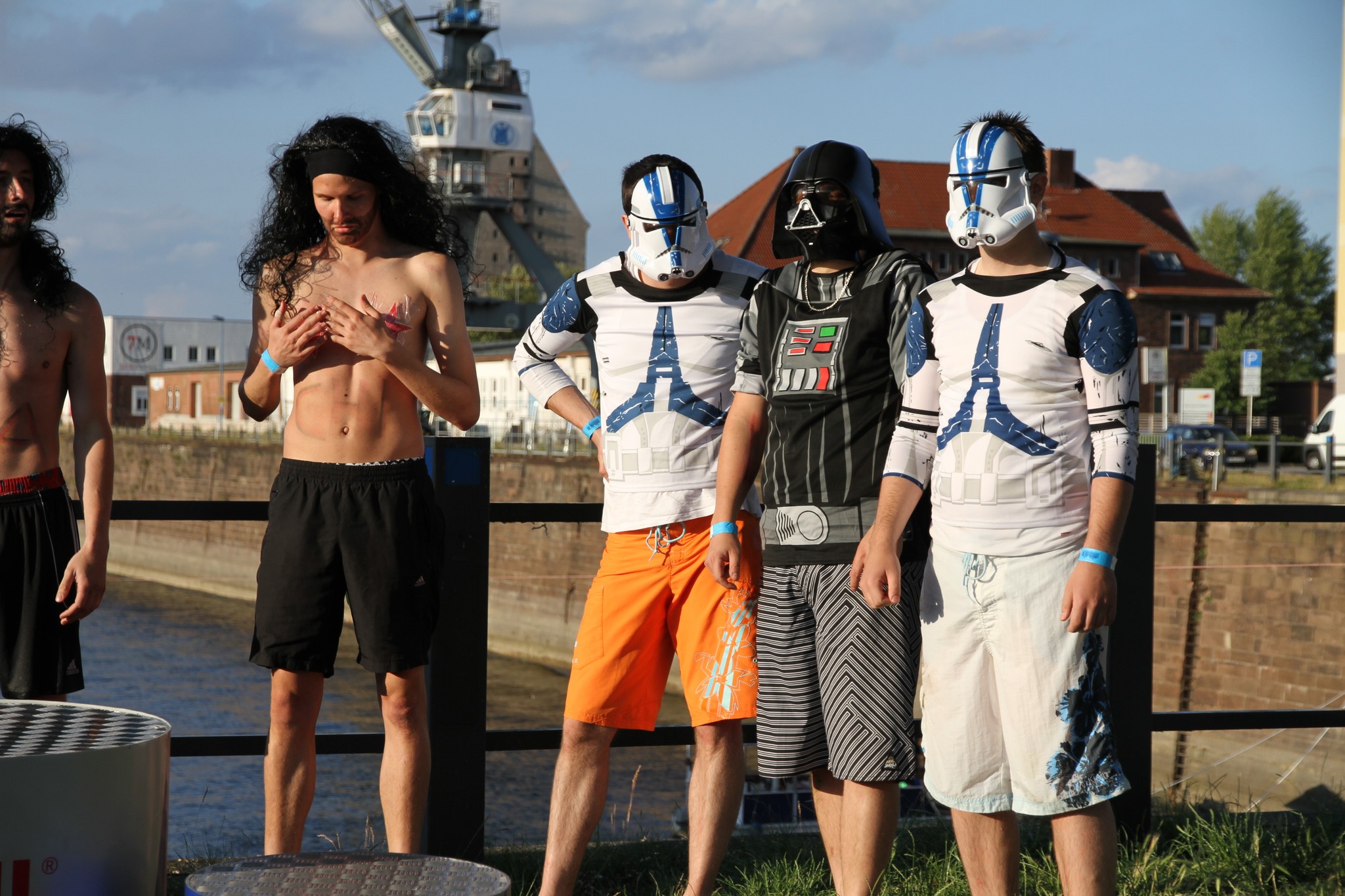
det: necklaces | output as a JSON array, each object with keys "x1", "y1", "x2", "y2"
[{"x1": 805, "y1": 262, "x2": 859, "y2": 311}]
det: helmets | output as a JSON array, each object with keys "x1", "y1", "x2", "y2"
[{"x1": 772, "y1": 140, "x2": 895, "y2": 258}]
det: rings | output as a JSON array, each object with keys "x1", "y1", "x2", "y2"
[{"x1": 274, "y1": 309, "x2": 285, "y2": 317}]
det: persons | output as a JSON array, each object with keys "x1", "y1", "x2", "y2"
[
  {"x1": 859, "y1": 110, "x2": 1140, "y2": 896},
  {"x1": 0, "y1": 110, "x2": 115, "y2": 704},
  {"x1": 704, "y1": 138, "x2": 941, "y2": 896},
  {"x1": 238, "y1": 114, "x2": 481, "y2": 856},
  {"x1": 512, "y1": 153, "x2": 768, "y2": 896}
]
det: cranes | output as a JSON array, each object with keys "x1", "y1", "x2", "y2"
[{"x1": 361, "y1": 0, "x2": 442, "y2": 90}]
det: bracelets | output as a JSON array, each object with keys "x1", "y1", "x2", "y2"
[
  {"x1": 582, "y1": 415, "x2": 601, "y2": 438},
  {"x1": 709, "y1": 522, "x2": 738, "y2": 538},
  {"x1": 261, "y1": 348, "x2": 289, "y2": 375},
  {"x1": 1077, "y1": 548, "x2": 1118, "y2": 571}
]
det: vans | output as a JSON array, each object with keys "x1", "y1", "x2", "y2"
[{"x1": 1303, "y1": 393, "x2": 1345, "y2": 469}]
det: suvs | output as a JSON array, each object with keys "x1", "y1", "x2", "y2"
[{"x1": 1162, "y1": 424, "x2": 1259, "y2": 476}]
